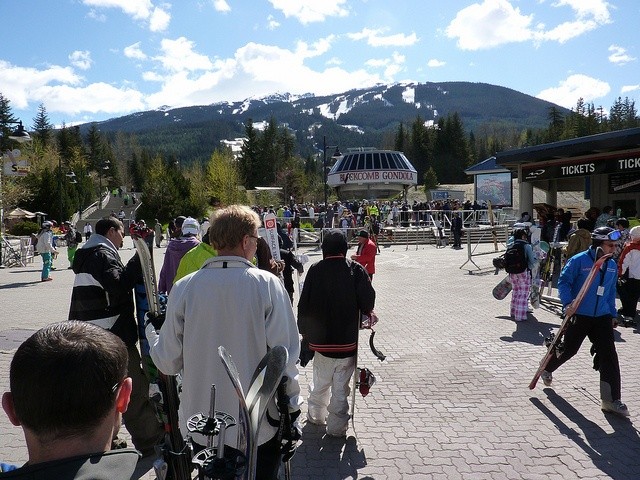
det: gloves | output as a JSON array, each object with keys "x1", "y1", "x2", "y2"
[
  {"x1": 280, "y1": 410, "x2": 301, "y2": 462},
  {"x1": 142, "y1": 312, "x2": 165, "y2": 334}
]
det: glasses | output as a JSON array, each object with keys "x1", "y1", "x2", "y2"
[
  {"x1": 608, "y1": 229, "x2": 622, "y2": 241},
  {"x1": 248, "y1": 233, "x2": 262, "y2": 244},
  {"x1": 602, "y1": 242, "x2": 618, "y2": 248}
]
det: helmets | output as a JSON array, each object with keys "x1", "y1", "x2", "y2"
[
  {"x1": 42, "y1": 220, "x2": 53, "y2": 227},
  {"x1": 174, "y1": 216, "x2": 185, "y2": 228},
  {"x1": 139, "y1": 220, "x2": 145, "y2": 226},
  {"x1": 203, "y1": 217, "x2": 209, "y2": 222},
  {"x1": 181, "y1": 216, "x2": 200, "y2": 236},
  {"x1": 493, "y1": 258, "x2": 505, "y2": 269},
  {"x1": 592, "y1": 226, "x2": 620, "y2": 250}
]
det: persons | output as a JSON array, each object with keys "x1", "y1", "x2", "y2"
[
  {"x1": 0, "y1": 320, "x2": 142, "y2": 479},
  {"x1": 50, "y1": 219, "x2": 60, "y2": 271},
  {"x1": 251, "y1": 202, "x2": 325, "y2": 229},
  {"x1": 154, "y1": 219, "x2": 164, "y2": 248},
  {"x1": 516, "y1": 212, "x2": 535, "y2": 226},
  {"x1": 536, "y1": 203, "x2": 571, "y2": 258},
  {"x1": 59, "y1": 222, "x2": 66, "y2": 245},
  {"x1": 172, "y1": 227, "x2": 258, "y2": 286},
  {"x1": 249, "y1": 236, "x2": 286, "y2": 276},
  {"x1": 36, "y1": 220, "x2": 59, "y2": 282},
  {"x1": 143, "y1": 205, "x2": 301, "y2": 480},
  {"x1": 297, "y1": 228, "x2": 378, "y2": 438},
  {"x1": 541, "y1": 226, "x2": 630, "y2": 417},
  {"x1": 118, "y1": 210, "x2": 126, "y2": 222},
  {"x1": 451, "y1": 212, "x2": 462, "y2": 249},
  {"x1": 277, "y1": 233, "x2": 304, "y2": 307},
  {"x1": 158, "y1": 217, "x2": 201, "y2": 298},
  {"x1": 595, "y1": 206, "x2": 618, "y2": 230},
  {"x1": 351, "y1": 230, "x2": 377, "y2": 281},
  {"x1": 112, "y1": 186, "x2": 123, "y2": 198},
  {"x1": 576, "y1": 207, "x2": 600, "y2": 233},
  {"x1": 504, "y1": 230, "x2": 534, "y2": 322},
  {"x1": 561, "y1": 218, "x2": 593, "y2": 271},
  {"x1": 408, "y1": 198, "x2": 451, "y2": 228},
  {"x1": 291, "y1": 217, "x2": 300, "y2": 249},
  {"x1": 325, "y1": 199, "x2": 409, "y2": 229},
  {"x1": 59, "y1": 221, "x2": 78, "y2": 269},
  {"x1": 84, "y1": 222, "x2": 92, "y2": 242},
  {"x1": 617, "y1": 226, "x2": 640, "y2": 318},
  {"x1": 131, "y1": 192, "x2": 137, "y2": 206},
  {"x1": 123, "y1": 193, "x2": 129, "y2": 206},
  {"x1": 110, "y1": 210, "x2": 117, "y2": 218},
  {"x1": 451, "y1": 198, "x2": 488, "y2": 220},
  {"x1": 613, "y1": 217, "x2": 631, "y2": 264},
  {"x1": 68, "y1": 216, "x2": 165, "y2": 458}
]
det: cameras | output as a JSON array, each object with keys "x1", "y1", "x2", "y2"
[{"x1": 364, "y1": 216, "x2": 371, "y2": 222}]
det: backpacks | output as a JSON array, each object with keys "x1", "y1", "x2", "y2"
[
  {"x1": 504, "y1": 242, "x2": 529, "y2": 274},
  {"x1": 74, "y1": 230, "x2": 82, "y2": 243}
]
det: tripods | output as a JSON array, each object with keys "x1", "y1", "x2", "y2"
[{"x1": 365, "y1": 222, "x2": 380, "y2": 255}]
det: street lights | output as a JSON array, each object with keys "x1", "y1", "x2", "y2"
[
  {"x1": 98, "y1": 159, "x2": 112, "y2": 208},
  {"x1": 58, "y1": 159, "x2": 78, "y2": 226},
  {"x1": 0, "y1": 120, "x2": 33, "y2": 143},
  {"x1": 62, "y1": 172, "x2": 77, "y2": 224},
  {"x1": 323, "y1": 135, "x2": 344, "y2": 211}
]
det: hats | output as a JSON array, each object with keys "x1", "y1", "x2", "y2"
[
  {"x1": 51, "y1": 220, "x2": 59, "y2": 227},
  {"x1": 63, "y1": 222, "x2": 71, "y2": 226},
  {"x1": 321, "y1": 228, "x2": 347, "y2": 255},
  {"x1": 356, "y1": 230, "x2": 368, "y2": 238},
  {"x1": 567, "y1": 228, "x2": 577, "y2": 240},
  {"x1": 629, "y1": 226, "x2": 640, "y2": 237}
]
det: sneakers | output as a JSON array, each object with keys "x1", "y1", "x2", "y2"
[
  {"x1": 526, "y1": 307, "x2": 533, "y2": 313},
  {"x1": 51, "y1": 268, "x2": 56, "y2": 270},
  {"x1": 540, "y1": 369, "x2": 552, "y2": 386},
  {"x1": 601, "y1": 399, "x2": 629, "y2": 416},
  {"x1": 43, "y1": 277, "x2": 53, "y2": 281},
  {"x1": 68, "y1": 267, "x2": 73, "y2": 269}
]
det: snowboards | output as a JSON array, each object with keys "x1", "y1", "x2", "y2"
[
  {"x1": 135, "y1": 249, "x2": 167, "y2": 380},
  {"x1": 528, "y1": 226, "x2": 541, "y2": 310},
  {"x1": 493, "y1": 242, "x2": 550, "y2": 300},
  {"x1": 293, "y1": 227, "x2": 301, "y2": 295},
  {"x1": 349, "y1": 311, "x2": 361, "y2": 442},
  {"x1": 263, "y1": 213, "x2": 284, "y2": 289}
]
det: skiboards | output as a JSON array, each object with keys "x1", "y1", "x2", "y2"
[
  {"x1": 134, "y1": 235, "x2": 186, "y2": 452},
  {"x1": 528, "y1": 247, "x2": 614, "y2": 389},
  {"x1": 218, "y1": 344, "x2": 290, "y2": 480}
]
